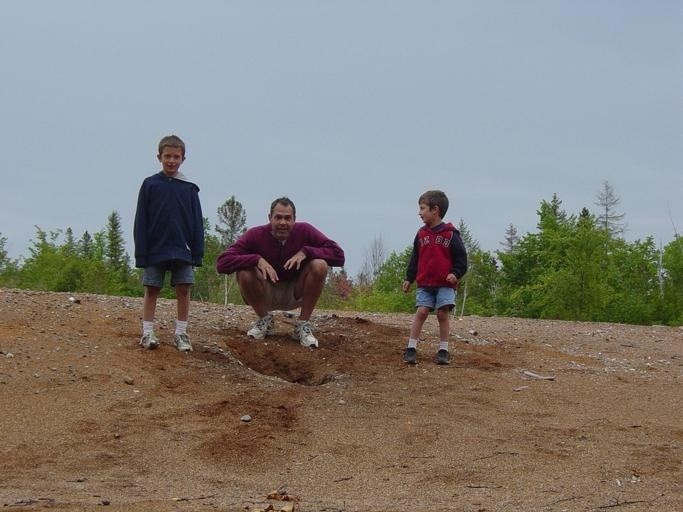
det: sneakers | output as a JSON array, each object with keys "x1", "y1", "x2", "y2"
[
  {"x1": 172, "y1": 331, "x2": 194, "y2": 350},
  {"x1": 247, "y1": 314, "x2": 275, "y2": 340},
  {"x1": 403, "y1": 347, "x2": 419, "y2": 364},
  {"x1": 139, "y1": 330, "x2": 161, "y2": 350},
  {"x1": 437, "y1": 348, "x2": 451, "y2": 364},
  {"x1": 290, "y1": 319, "x2": 319, "y2": 349}
]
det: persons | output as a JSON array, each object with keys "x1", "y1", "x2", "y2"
[
  {"x1": 399, "y1": 191, "x2": 468, "y2": 364},
  {"x1": 133, "y1": 135, "x2": 204, "y2": 352},
  {"x1": 215, "y1": 195, "x2": 344, "y2": 348}
]
